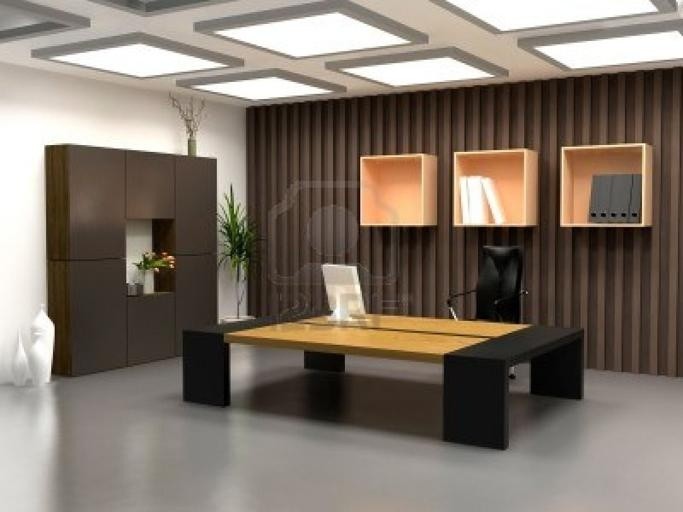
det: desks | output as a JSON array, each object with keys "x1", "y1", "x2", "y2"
[{"x1": 182, "y1": 310, "x2": 585, "y2": 451}]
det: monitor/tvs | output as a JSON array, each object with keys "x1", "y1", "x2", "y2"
[{"x1": 321, "y1": 262, "x2": 367, "y2": 323}]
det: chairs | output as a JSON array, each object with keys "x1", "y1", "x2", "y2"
[{"x1": 446, "y1": 245, "x2": 529, "y2": 380}]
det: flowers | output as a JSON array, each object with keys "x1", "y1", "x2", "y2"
[{"x1": 133, "y1": 251, "x2": 175, "y2": 273}]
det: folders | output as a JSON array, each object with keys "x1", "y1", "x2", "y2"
[
  {"x1": 587, "y1": 172, "x2": 640, "y2": 223},
  {"x1": 459, "y1": 175, "x2": 506, "y2": 225}
]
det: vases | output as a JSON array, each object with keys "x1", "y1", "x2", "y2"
[
  {"x1": 143, "y1": 271, "x2": 154, "y2": 294},
  {"x1": 12, "y1": 304, "x2": 55, "y2": 387}
]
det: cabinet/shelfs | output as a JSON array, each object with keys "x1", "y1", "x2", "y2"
[
  {"x1": 359, "y1": 153, "x2": 438, "y2": 226},
  {"x1": 559, "y1": 142, "x2": 653, "y2": 228},
  {"x1": 452, "y1": 148, "x2": 541, "y2": 228}
]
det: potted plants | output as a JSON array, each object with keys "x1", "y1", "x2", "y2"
[
  {"x1": 214, "y1": 182, "x2": 267, "y2": 325},
  {"x1": 168, "y1": 91, "x2": 210, "y2": 158}
]
